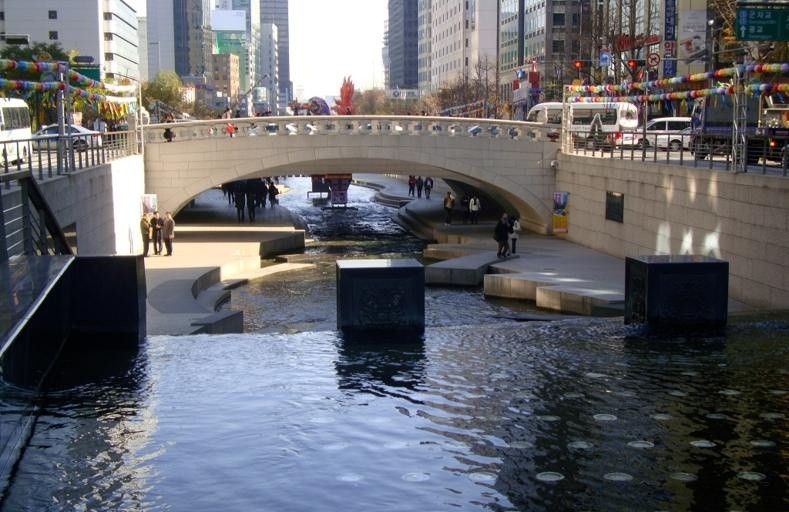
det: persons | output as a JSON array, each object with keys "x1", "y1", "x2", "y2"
[
  {"x1": 150, "y1": 211, "x2": 164, "y2": 255},
  {"x1": 443, "y1": 191, "x2": 481, "y2": 226},
  {"x1": 408, "y1": 174, "x2": 433, "y2": 199},
  {"x1": 160, "y1": 112, "x2": 175, "y2": 142},
  {"x1": 346, "y1": 108, "x2": 351, "y2": 128},
  {"x1": 493, "y1": 212, "x2": 514, "y2": 258},
  {"x1": 222, "y1": 177, "x2": 279, "y2": 224},
  {"x1": 217, "y1": 107, "x2": 272, "y2": 119},
  {"x1": 140, "y1": 213, "x2": 150, "y2": 257},
  {"x1": 161, "y1": 212, "x2": 175, "y2": 256}
]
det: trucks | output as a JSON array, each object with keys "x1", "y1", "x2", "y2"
[{"x1": 690, "y1": 91, "x2": 788, "y2": 165}]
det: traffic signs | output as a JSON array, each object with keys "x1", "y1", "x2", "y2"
[{"x1": 734, "y1": 6, "x2": 789, "y2": 42}]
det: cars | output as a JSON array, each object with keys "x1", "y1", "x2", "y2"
[
  {"x1": 31, "y1": 124, "x2": 103, "y2": 152},
  {"x1": 653, "y1": 126, "x2": 691, "y2": 152}
]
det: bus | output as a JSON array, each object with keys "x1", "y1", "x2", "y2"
[
  {"x1": 0, "y1": 98, "x2": 34, "y2": 167},
  {"x1": 526, "y1": 100, "x2": 639, "y2": 152}
]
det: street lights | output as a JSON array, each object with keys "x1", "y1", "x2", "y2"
[
  {"x1": 707, "y1": 19, "x2": 716, "y2": 89},
  {"x1": 57, "y1": 60, "x2": 70, "y2": 175}
]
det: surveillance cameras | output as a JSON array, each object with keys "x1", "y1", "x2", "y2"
[{"x1": 551, "y1": 160, "x2": 555, "y2": 168}]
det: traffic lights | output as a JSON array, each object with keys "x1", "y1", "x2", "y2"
[
  {"x1": 629, "y1": 59, "x2": 646, "y2": 68},
  {"x1": 575, "y1": 60, "x2": 593, "y2": 69}
]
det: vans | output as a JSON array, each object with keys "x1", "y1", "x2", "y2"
[{"x1": 638, "y1": 117, "x2": 693, "y2": 152}]
